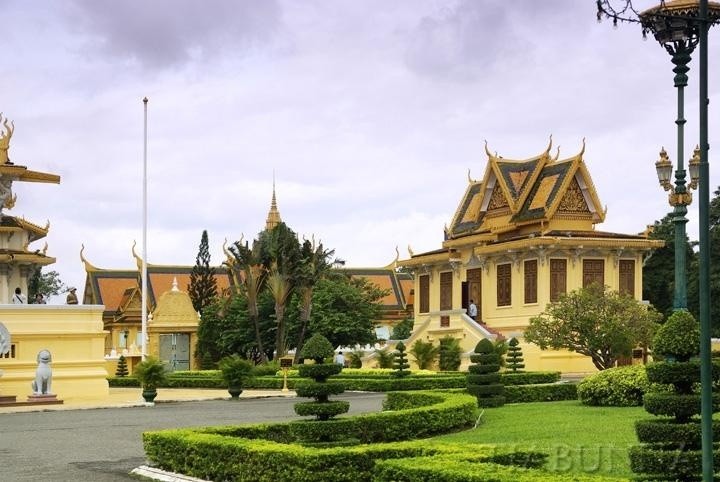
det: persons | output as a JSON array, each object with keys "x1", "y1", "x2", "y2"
[
  {"x1": 468, "y1": 300, "x2": 477, "y2": 320},
  {"x1": 12, "y1": 286, "x2": 78, "y2": 304},
  {"x1": 335, "y1": 351, "x2": 345, "y2": 366}
]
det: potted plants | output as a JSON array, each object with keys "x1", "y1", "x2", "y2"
[
  {"x1": 213, "y1": 353, "x2": 253, "y2": 398},
  {"x1": 133, "y1": 353, "x2": 176, "y2": 405}
]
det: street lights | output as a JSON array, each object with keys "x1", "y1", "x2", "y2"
[
  {"x1": 638, "y1": 3, "x2": 720, "y2": 362},
  {"x1": 124, "y1": 327, "x2": 130, "y2": 349},
  {"x1": 592, "y1": 0, "x2": 719, "y2": 482}
]
